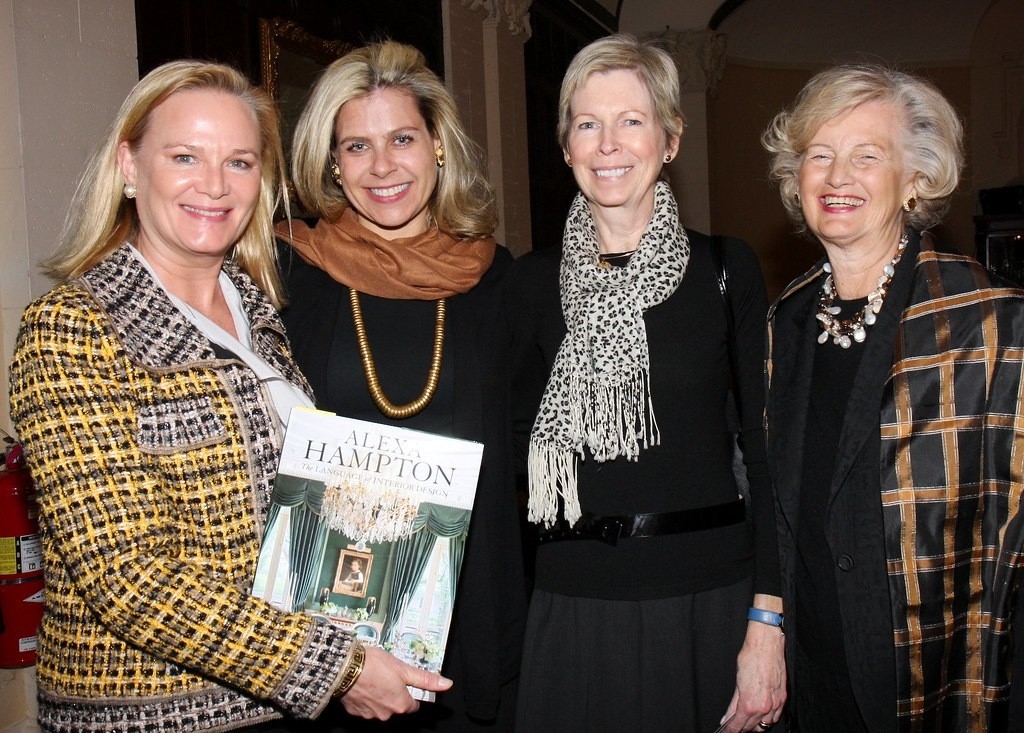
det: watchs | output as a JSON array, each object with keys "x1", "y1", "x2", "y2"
[{"x1": 747, "y1": 606, "x2": 786, "y2": 633}]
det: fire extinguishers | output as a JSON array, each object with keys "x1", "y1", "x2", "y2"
[{"x1": 0, "y1": 428, "x2": 48, "y2": 669}]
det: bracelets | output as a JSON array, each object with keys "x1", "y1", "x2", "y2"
[{"x1": 330, "y1": 638, "x2": 366, "y2": 701}]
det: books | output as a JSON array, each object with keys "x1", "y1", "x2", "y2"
[{"x1": 251, "y1": 404, "x2": 485, "y2": 705}]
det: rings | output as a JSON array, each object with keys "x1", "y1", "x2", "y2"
[{"x1": 759, "y1": 720, "x2": 772, "y2": 730}]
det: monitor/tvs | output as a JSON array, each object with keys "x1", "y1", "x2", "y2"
[{"x1": 985, "y1": 234, "x2": 1024, "y2": 288}]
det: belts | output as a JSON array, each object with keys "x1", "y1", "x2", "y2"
[{"x1": 541, "y1": 497, "x2": 751, "y2": 544}]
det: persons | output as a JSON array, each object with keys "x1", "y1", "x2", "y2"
[
  {"x1": 6, "y1": 59, "x2": 459, "y2": 732},
  {"x1": 727, "y1": 58, "x2": 1023, "y2": 733},
  {"x1": 485, "y1": 32, "x2": 791, "y2": 733},
  {"x1": 255, "y1": 36, "x2": 551, "y2": 733}
]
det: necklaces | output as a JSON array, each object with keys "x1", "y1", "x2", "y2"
[
  {"x1": 814, "y1": 231, "x2": 909, "y2": 350},
  {"x1": 349, "y1": 286, "x2": 447, "y2": 421},
  {"x1": 599, "y1": 252, "x2": 636, "y2": 261}
]
